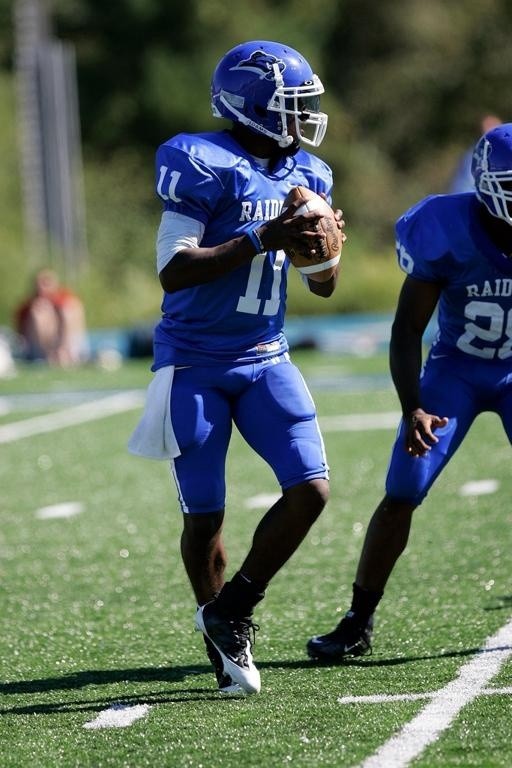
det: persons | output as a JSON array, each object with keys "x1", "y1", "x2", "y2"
[
  {"x1": 152, "y1": 38, "x2": 350, "y2": 700},
  {"x1": 11, "y1": 266, "x2": 92, "y2": 368},
  {"x1": 305, "y1": 119, "x2": 512, "y2": 666}
]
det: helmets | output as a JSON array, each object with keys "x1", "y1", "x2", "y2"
[
  {"x1": 210, "y1": 40, "x2": 329, "y2": 149},
  {"x1": 469, "y1": 122, "x2": 511, "y2": 227}
]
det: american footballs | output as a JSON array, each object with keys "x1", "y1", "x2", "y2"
[{"x1": 281, "y1": 185, "x2": 342, "y2": 282}]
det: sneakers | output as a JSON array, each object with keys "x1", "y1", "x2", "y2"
[
  {"x1": 190, "y1": 572, "x2": 265, "y2": 699},
  {"x1": 306, "y1": 582, "x2": 378, "y2": 658}
]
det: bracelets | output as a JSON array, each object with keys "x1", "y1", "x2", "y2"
[{"x1": 246, "y1": 229, "x2": 265, "y2": 252}]
